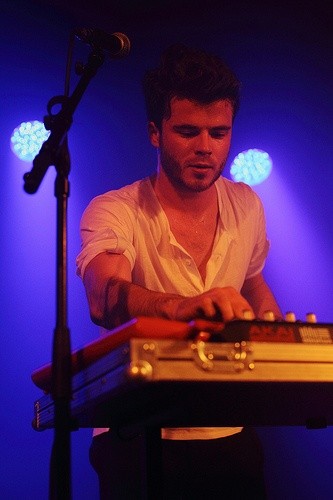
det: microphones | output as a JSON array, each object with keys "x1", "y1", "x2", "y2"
[{"x1": 78, "y1": 26, "x2": 131, "y2": 60}]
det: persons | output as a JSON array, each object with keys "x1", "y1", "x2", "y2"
[{"x1": 74, "y1": 38, "x2": 285, "y2": 500}]
